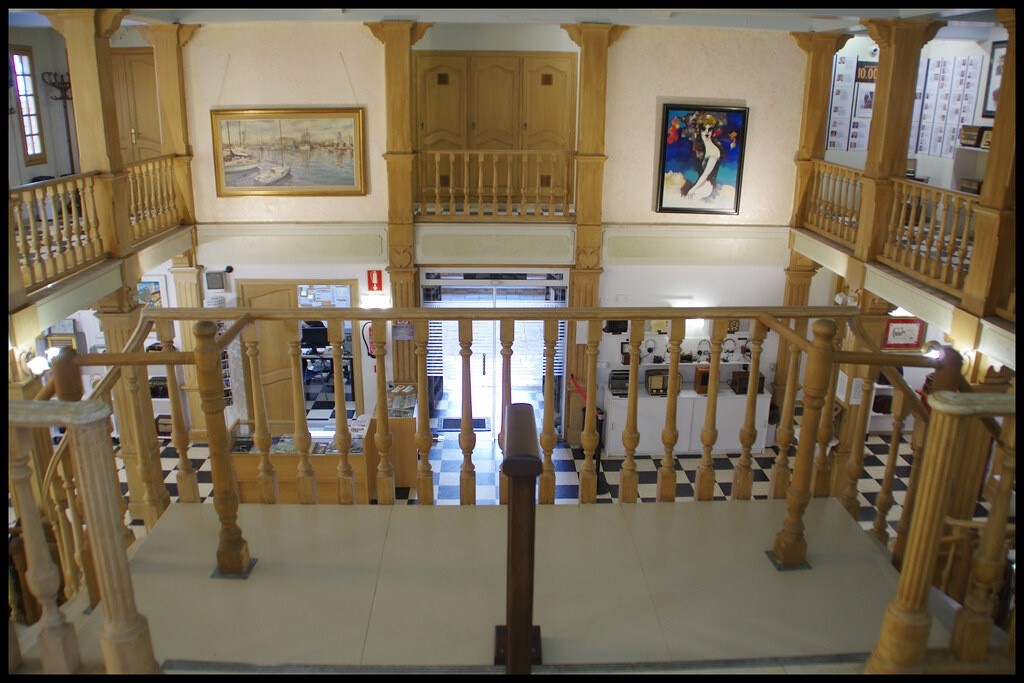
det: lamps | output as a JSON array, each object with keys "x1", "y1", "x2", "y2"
[{"x1": 25, "y1": 347, "x2": 61, "y2": 380}]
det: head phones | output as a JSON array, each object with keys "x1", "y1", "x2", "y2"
[
  {"x1": 722, "y1": 339, "x2": 736, "y2": 353},
  {"x1": 697, "y1": 339, "x2": 712, "y2": 355}
]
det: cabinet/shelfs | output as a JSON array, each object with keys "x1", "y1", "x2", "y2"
[
  {"x1": 602, "y1": 332, "x2": 772, "y2": 457},
  {"x1": 103, "y1": 350, "x2": 190, "y2": 439},
  {"x1": 865, "y1": 382, "x2": 911, "y2": 433},
  {"x1": 900, "y1": 176, "x2": 930, "y2": 225},
  {"x1": 227, "y1": 383, "x2": 418, "y2": 504},
  {"x1": 945, "y1": 148, "x2": 989, "y2": 238}
]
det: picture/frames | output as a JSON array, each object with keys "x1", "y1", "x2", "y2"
[
  {"x1": 882, "y1": 316, "x2": 928, "y2": 351},
  {"x1": 91, "y1": 275, "x2": 169, "y2": 339},
  {"x1": 982, "y1": 40, "x2": 1010, "y2": 118},
  {"x1": 655, "y1": 103, "x2": 749, "y2": 215},
  {"x1": 211, "y1": 107, "x2": 366, "y2": 198}
]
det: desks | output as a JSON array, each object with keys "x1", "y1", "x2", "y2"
[{"x1": 301, "y1": 353, "x2": 355, "y2": 401}]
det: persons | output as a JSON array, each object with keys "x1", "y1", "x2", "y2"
[{"x1": 300, "y1": 320, "x2": 326, "y2": 344}]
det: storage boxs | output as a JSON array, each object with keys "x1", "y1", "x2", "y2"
[
  {"x1": 146, "y1": 343, "x2": 175, "y2": 352},
  {"x1": 154, "y1": 415, "x2": 173, "y2": 435},
  {"x1": 148, "y1": 377, "x2": 169, "y2": 398}
]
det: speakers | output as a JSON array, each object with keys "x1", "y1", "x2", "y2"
[
  {"x1": 607, "y1": 320, "x2": 628, "y2": 335},
  {"x1": 728, "y1": 320, "x2": 740, "y2": 334}
]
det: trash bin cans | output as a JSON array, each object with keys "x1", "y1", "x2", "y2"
[{"x1": 31, "y1": 175, "x2": 59, "y2": 221}]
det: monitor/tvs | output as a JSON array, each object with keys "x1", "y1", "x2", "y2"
[{"x1": 302, "y1": 327, "x2": 327, "y2": 354}]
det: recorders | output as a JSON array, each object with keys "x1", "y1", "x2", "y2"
[
  {"x1": 609, "y1": 370, "x2": 630, "y2": 397},
  {"x1": 731, "y1": 371, "x2": 765, "y2": 394},
  {"x1": 695, "y1": 366, "x2": 711, "y2": 394},
  {"x1": 645, "y1": 369, "x2": 682, "y2": 397}
]
